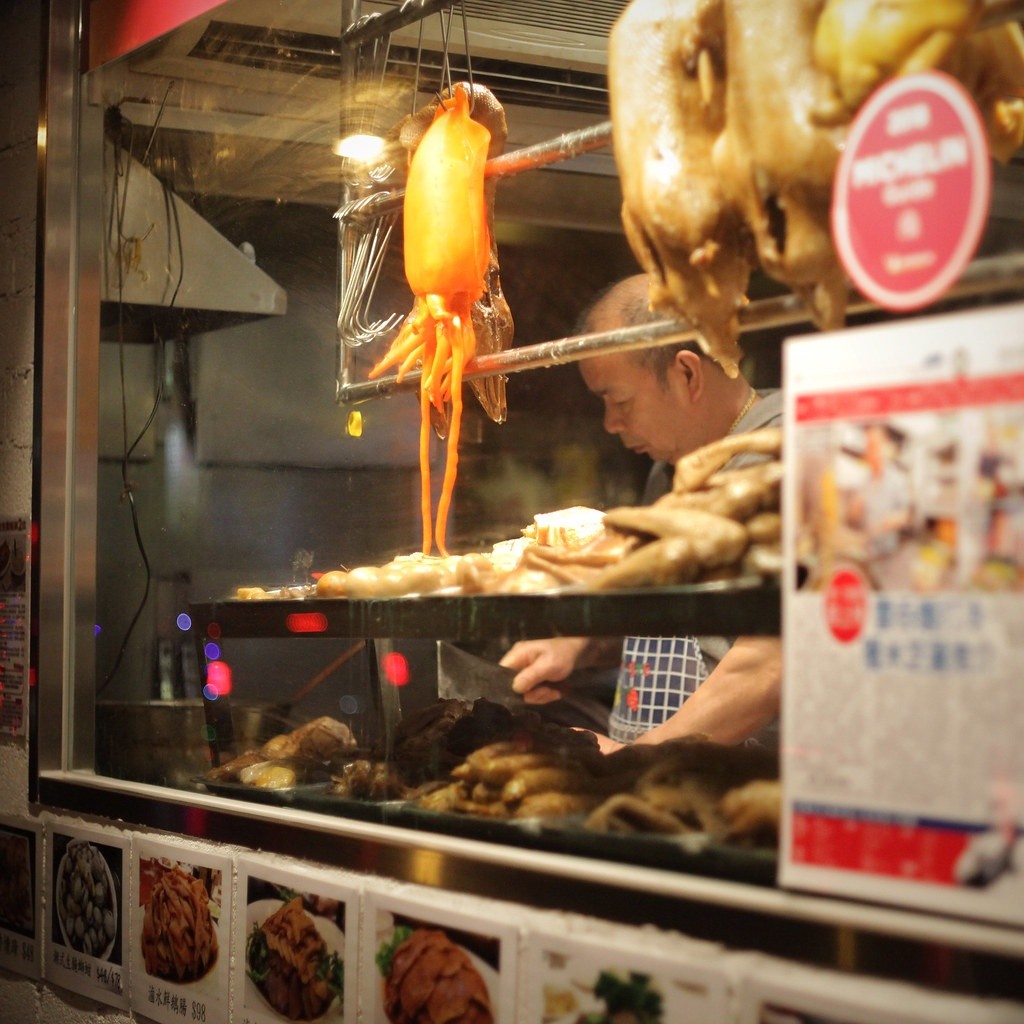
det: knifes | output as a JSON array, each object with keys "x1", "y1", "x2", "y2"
[{"x1": 437, "y1": 640, "x2": 523, "y2": 709}]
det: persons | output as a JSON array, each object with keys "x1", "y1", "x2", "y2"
[{"x1": 499, "y1": 264, "x2": 781, "y2": 784}]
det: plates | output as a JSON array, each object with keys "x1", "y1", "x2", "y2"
[
  {"x1": 378, "y1": 931, "x2": 501, "y2": 1024},
  {"x1": 246, "y1": 898, "x2": 344, "y2": 1024},
  {"x1": 137, "y1": 905, "x2": 219, "y2": 996},
  {"x1": 57, "y1": 837, "x2": 117, "y2": 961}
]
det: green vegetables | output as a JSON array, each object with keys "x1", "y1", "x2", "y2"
[
  {"x1": 577, "y1": 969, "x2": 663, "y2": 1024},
  {"x1": 375, "y1": 925, "x2": 412, "y2": 977},
  {"x1": 312, "y1": 950, "x2": 344, "y2": 995},
  {"x1": 245, "y1": 922, "x2": 273, "y2": 984}
]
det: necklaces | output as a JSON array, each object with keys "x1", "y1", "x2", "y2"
[{"x1": 726, "y1": 388, "x2": 757, "y2": 439}]
